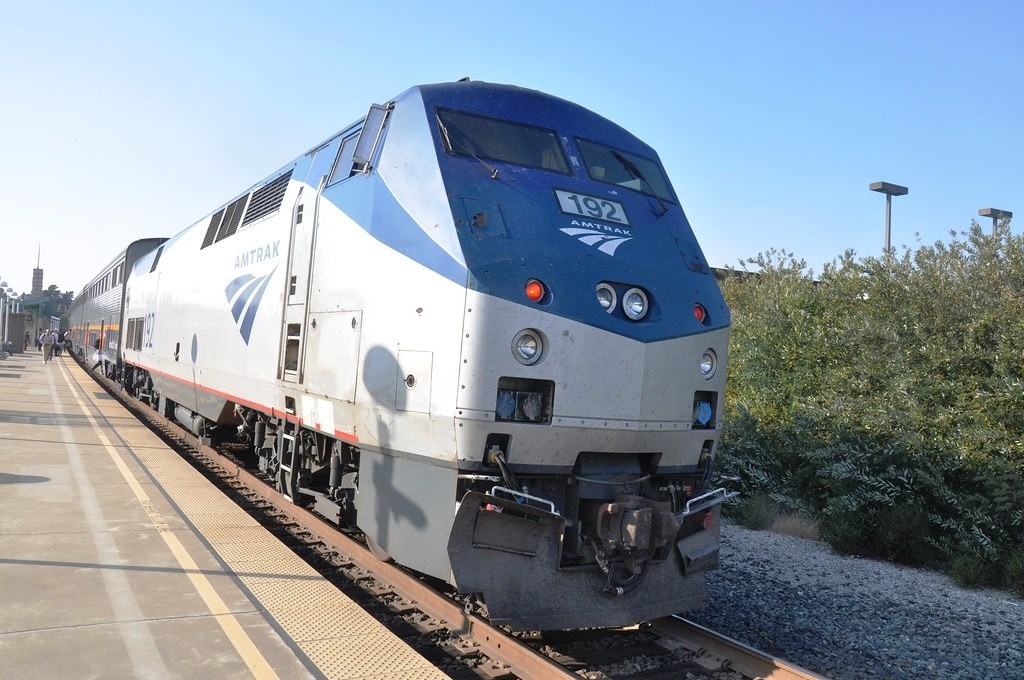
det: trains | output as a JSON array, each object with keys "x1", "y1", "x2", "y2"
[{"x1": 67, "y1": 77, "x2": 731, "y2": 631}]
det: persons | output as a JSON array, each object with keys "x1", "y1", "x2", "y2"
[
  {"x1": 88, "y1": 333, "x2": 102, "y2": 374},
  {"x1": 34, "y1": 328, "x2": 72, "y2": 360},
  {"x1": 39, "y1": 329, "x2": 54, "y2": 364},
  {"x1": 24, "y1": 331, "x2": 30, "y2": 350}
]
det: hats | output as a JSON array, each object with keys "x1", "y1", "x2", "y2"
[{"x1": 55, "y1": 328, "x2": 59, "y2": 331}]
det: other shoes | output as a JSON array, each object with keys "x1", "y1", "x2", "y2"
[{"x1": 44, "y1": 360, "x2": 47, "y2": 364}]
